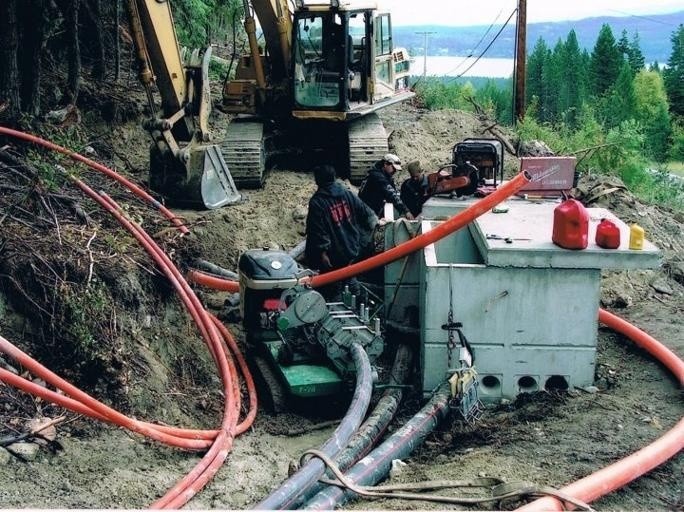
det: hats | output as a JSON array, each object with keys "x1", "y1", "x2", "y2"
[{"x1": 382, "y1": 154, "x2": 402, "y2": 171}]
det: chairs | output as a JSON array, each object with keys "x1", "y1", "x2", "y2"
[{"x1": 314, "y1": 33, "x2": 353, "y2": 95}]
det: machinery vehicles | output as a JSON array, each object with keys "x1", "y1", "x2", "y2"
[
  {"x1": 230, "y1": 248, "x2": 392, "y2": 418},
  {"x1": 120, "y1": 0, "x2": 417, "y2": 213}
]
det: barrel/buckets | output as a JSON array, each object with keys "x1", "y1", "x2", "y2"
[{"x1": 552, "y1": 194, "x2": 591, "y2": 250}]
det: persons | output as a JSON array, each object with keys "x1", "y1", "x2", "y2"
[
  {"x1": 397, "y1": 162, "x2": 433, "y2": 223},
  {"x1": 304, "y1": 160, "x2": 381, "y2": 300},
  {"x1": 361, "y1": 151, "x2": 414, "y2": 225}
]
